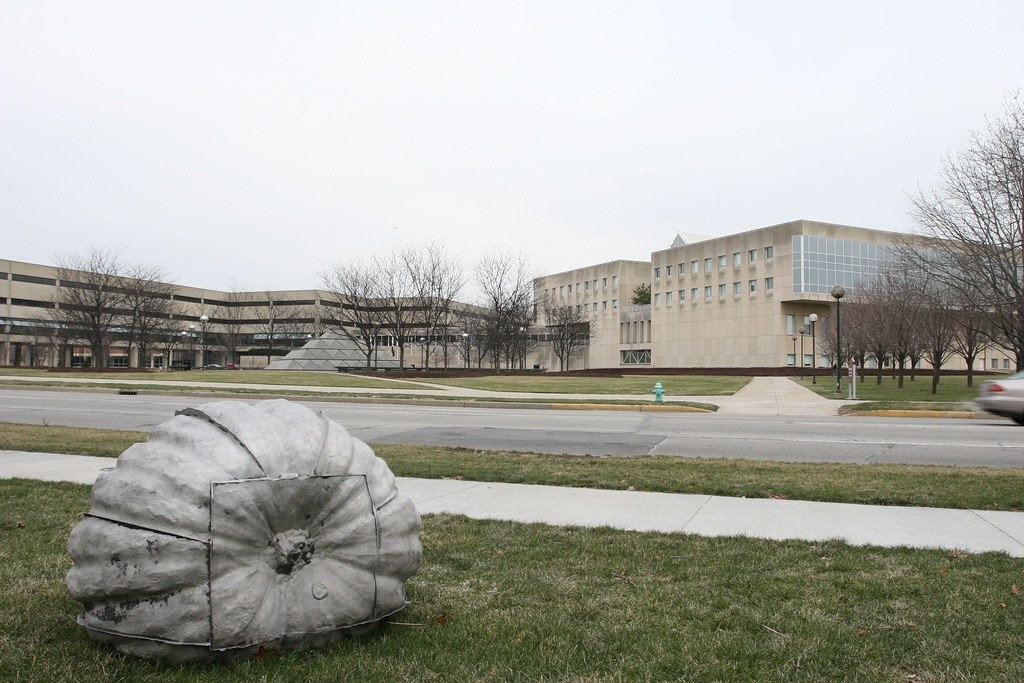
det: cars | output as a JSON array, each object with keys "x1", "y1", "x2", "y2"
[
  {"x1": 225, "y1": 363, "x2": 239, "y2": 370},
  {"x1": 204, "y1": 364, "x2": 221, "y2": 370},
  {"x1": 975, "y1": 368, "x2": 1024, "y2": 426}
]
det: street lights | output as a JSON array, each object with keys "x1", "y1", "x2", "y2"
[
  {"x1": 188, "y1": 326, "x2": 195, "y2": 370},
  {"x1": 798, "y1": 327, "x2": 806, "y2": 379},
  {"x1": 462, "y1": 332, "x2": 468, "y2": 368},
  {"x1": 831, "y1": 286, "x2": 846, "y2": 393},
  {"x1": 180, "y1": 331, "x2": 188, "y2": 366},
  {"x1": 809, "y1": 313, "x2": 818, "y2": 384},
  {"x1": 199, "y1": 315, "x2": 209, "y2": 370},
  {"x1": 792, "y1": 336, "x2": 797, "y2": 367},
  {"x1": 419, "y1": 337, "x2": 425, "y2": 369}
]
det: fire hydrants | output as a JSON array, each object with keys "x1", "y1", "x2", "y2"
[{"x1": 651, "y1": 383, "x2": 666, "y2": 402}]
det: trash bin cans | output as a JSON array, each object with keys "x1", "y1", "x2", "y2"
[
  {"x1": 184, "y1": 359, "x2": 192, "y2": 370},
  {"x1": 534, "y1": 365, "x2": 539, "y2": 369}
]
